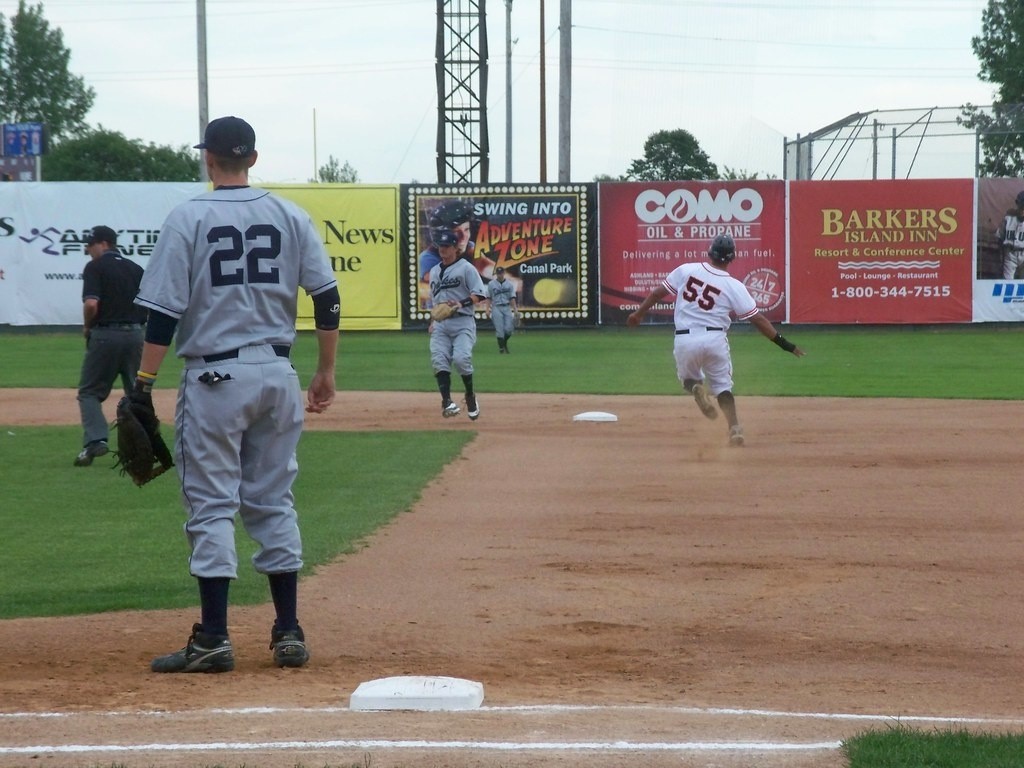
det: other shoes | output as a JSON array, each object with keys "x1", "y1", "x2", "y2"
[
  {"x1": 497, "y1": 337, "x2": 505, "y2": 353},
  {"x1": 504, "y1": 333, "x2": 510, "y2": 348},
  {"x1": 727, "y1": 425, "x2": 746, "y2": 447},
  {"x1": 441, "y1": 398, "x2": 461, "y2": 418},
  {"x1": 460, "y1": 391, "x2": 480, "y2": 419},
  {"x1": 691, "y1": 383, "x2": 718, "y2": 420},
  {"x1": 73, "y1": 440, "x2": 110, "y2": 467}
]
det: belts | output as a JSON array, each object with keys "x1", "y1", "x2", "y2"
[
  {"x1": 93, "y1": 323, "x2": 143, "y2": 331},
  {"x1": 202, "y1": 345, "x2": 290, "y2": 361},
  {"x1": 676, "y1": 327, "x2": 723, "y2": 334}
]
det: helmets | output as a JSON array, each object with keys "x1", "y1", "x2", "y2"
[
  {"x1": 707, "y1": 233, "x2": 736, "y2": 263},
  {"x1": 429, "y1": 199, "x2": 474, "y2": 249}
]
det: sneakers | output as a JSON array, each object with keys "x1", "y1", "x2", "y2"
[
  {"x1": 151, "y1": 623, "x2": 235, "y2": 674},
  {"x1": 268, "y1": 618, "x2": 311, "y2": 667}
]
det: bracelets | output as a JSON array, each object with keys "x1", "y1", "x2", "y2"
[{"x1": 138, "y1": 370, "x2": 158, "y2": 379}]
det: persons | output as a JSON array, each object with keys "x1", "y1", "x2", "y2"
[
  {"x1": 486, "y1": 267, "x2": 518, "y2": 355},
  {"x1": 428, "y1": 230, "x2": 487, "y2": 421},
  {"x1": 417, "y1": 200, "x2": 522, "y2": 311},
  {"x1": 76, "y1": 226, "x2": 148, "y2": 467},
  {"x1": 627, "y1": 234, "x2": 806, "y2": 446},
  {"x1": 131, "y1": 117, "x2": 341, "y2": 674},
  {"x1": 994, "y1": 191, "x2": 1024, "y2": 279}
]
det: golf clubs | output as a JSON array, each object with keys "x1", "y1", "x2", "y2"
[{"x1": 117, "y1": 390, "x2": 173, "y2": 488}]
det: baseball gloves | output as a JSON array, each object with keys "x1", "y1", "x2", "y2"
[
  {"x1": 429, "y1": 302, "x2": 457, "y2": 322},
  {"x1": 512, "y1": 311, "x2": 522, "y2": 328}
]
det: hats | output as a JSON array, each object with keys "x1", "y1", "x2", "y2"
[
  {"x1": 192, "y1": 116, "x2": 256, "y2": 159},
  {"x1": 437, "y1": 231, "x2": 459, "y2": 246},
  {"x1": 494, "y1": 267, "x2": 504, "y2": 274},
  {"x1": 80, "y1": 226, "x2": 119, "y2": 244}
]
